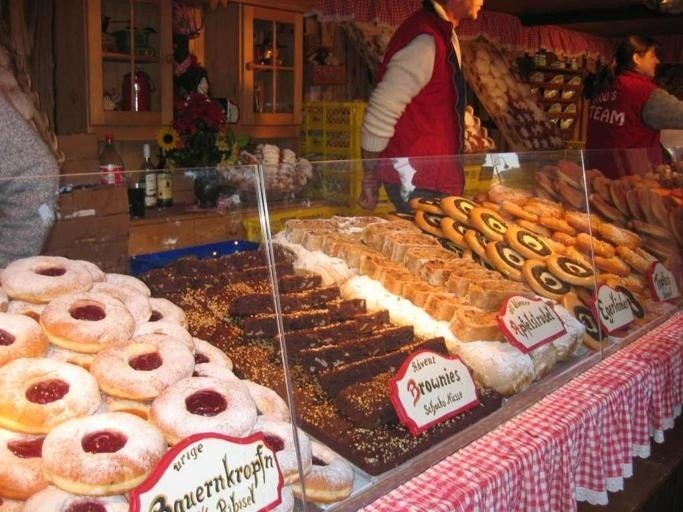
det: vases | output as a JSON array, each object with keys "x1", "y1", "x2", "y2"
[{"x1": 192, "y1": 165, "x2": 219, "y2": 209}]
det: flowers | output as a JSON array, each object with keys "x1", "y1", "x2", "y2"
[{"x1": 157, "y1": 94, "x2": 249, "y2": 173}]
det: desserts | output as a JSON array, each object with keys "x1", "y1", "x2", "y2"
[
  {"x1": 142, "y1": 237, "x2": 504, "y2": 473},
  {"x1": 279, "y1": 157, "x2": 678, "y2": 393}
]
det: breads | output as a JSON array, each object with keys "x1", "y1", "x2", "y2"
[{"x1": 463, "y1": 43, "x2": 564, "y2": 153}]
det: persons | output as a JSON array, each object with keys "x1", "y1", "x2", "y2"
[
  {"x1": 582, "y1": 32, "x2": 683, "y2": 180},
  {"x1": 355, "y1": 0, "x2": 485, "y2": 218}
]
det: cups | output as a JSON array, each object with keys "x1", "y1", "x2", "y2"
[{"x1": 125, "y1": 181, "x2": 146, "y2": 219}]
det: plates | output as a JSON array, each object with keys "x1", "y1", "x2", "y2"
[{"x1": 529, "y1": 71, "x2": 581, "y2": 129}]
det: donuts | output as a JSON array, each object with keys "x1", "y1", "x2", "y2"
[{"x1": 1, "y1": 256, "x2": 354, "y2": 511}]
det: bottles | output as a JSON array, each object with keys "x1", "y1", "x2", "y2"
[
  {"x1": 260, "y1": 30, "x2": 273, "y2": 64},
  {"x1": 100, "y1": 134, "x2": 123, "y2": 186},
  {"x1": 141, "y1": 144, "x2": 176, "y2": 206},
  {"x1": 123, "y1": 70, "x2": 154, "y2": 111}
]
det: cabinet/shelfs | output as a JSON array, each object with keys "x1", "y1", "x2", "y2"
[
  {"x1": 204, "y1": 4, "x2": 305, "y2": 141},
  {"x1": 0, "y1": 144, "x2": 683, "y2": 512},
  {"x1": 54, "y1": 0, "x2": 173, "y2": 138},
  {"x1": 128, "y1": 196, "x2": 333, "y2": 255}
]
